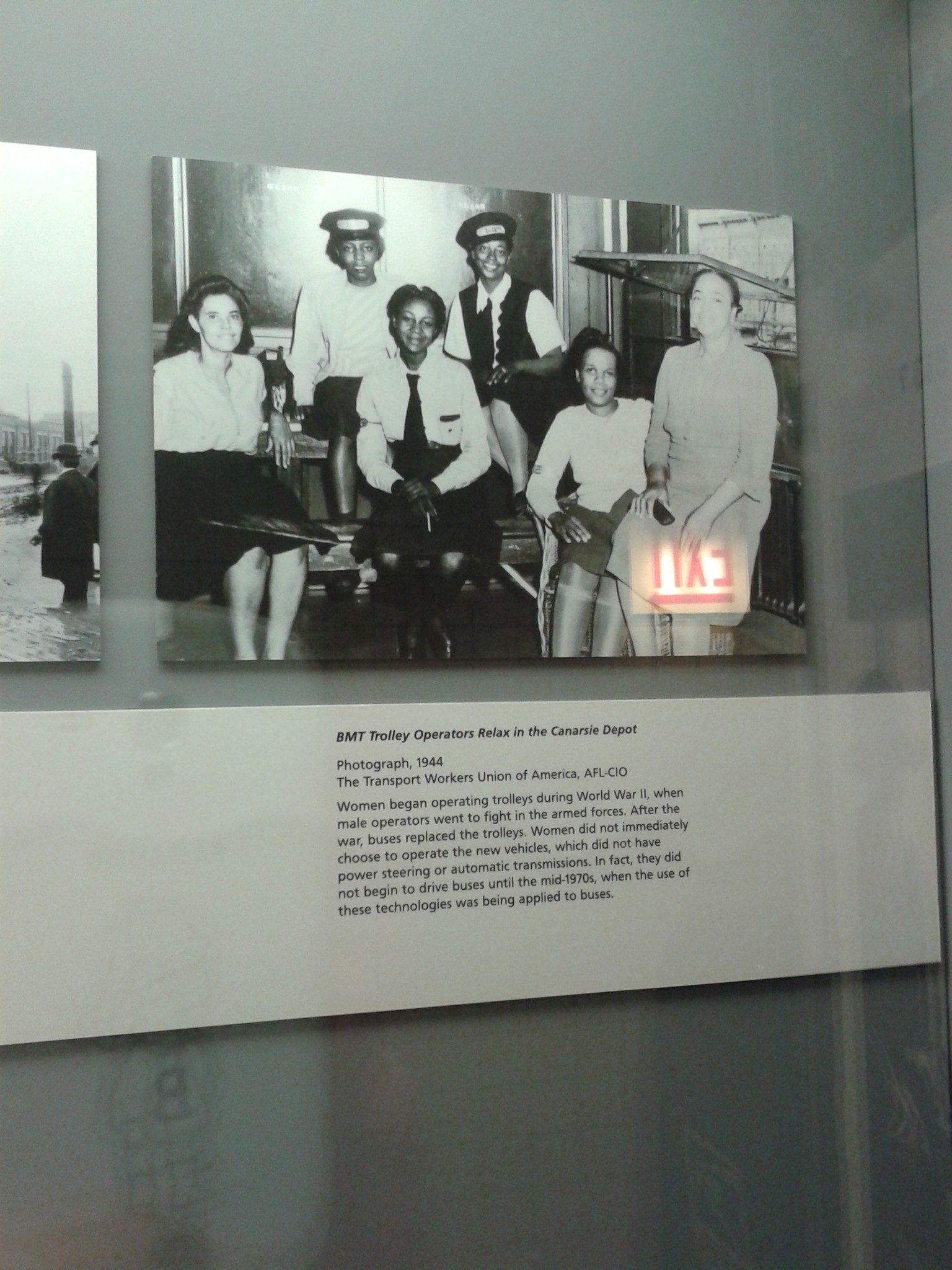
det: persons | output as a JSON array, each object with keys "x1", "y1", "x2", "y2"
[{"x1": 29, "y1": 208, "x2": 780, "y2": 654}]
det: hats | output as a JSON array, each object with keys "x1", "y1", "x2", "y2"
[
  {"x1": 51, "y1": 443, "x2": 82, "y2": 457},
  {"x1": 320, "y1": 209, "x2": 386, "y2": 243},
  {"x1": 91, "y1": 434, "x2": 99, "y2": 447},
  {"x1": 461, "y1": 212, "x2": 517, "y2": 249}
]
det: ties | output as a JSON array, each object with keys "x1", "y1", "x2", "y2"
[{"x1": 403, "y1": 374, "x2": 429, "y2": 463}]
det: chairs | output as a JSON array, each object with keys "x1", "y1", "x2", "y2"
[{"x1": 538, "y1": 491, "x2": 735, "y2": 657}]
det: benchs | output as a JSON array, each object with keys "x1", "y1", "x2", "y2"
[{"x1": 256, "y1": 433, "x2": 542, "y2": 572}]
